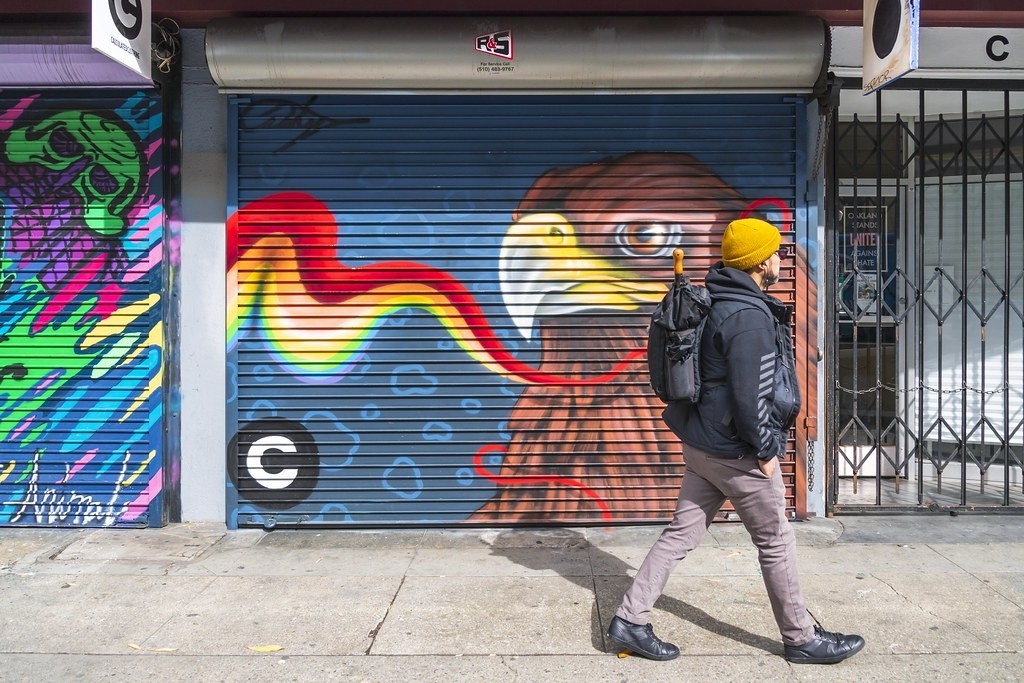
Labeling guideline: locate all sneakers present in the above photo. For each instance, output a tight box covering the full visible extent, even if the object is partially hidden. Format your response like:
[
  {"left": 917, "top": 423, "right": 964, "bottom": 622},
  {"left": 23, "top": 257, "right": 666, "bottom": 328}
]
[
  {"left": 607, "top": 616, "right": 680, "bottom": 662},
  {"left": 785, "top": 629, "right": 864, "bottom": 664}
]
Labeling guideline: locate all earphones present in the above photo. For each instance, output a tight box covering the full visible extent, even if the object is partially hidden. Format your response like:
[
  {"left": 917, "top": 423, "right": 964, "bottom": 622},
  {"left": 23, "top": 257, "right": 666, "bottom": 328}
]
[{"left": 761, "top": 261, "right": 766, "bottom": 267}]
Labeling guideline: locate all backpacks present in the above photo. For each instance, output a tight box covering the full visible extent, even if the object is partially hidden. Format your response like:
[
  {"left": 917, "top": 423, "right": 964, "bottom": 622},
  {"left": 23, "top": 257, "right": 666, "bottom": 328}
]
[{"left": 646, "top": 278, "right": 774, "bottom": 406}]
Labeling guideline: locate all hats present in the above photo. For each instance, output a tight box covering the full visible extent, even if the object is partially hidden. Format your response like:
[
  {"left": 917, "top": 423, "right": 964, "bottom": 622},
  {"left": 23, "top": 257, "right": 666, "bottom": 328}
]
[{"left": 721, "top": 219, "right": 781, "bottom": 271}]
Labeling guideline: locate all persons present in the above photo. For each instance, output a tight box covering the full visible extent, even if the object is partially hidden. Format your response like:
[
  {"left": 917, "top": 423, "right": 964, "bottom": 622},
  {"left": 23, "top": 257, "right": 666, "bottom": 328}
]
[{"left": 606, "top": 219, "right": 866, "bottom": 664}]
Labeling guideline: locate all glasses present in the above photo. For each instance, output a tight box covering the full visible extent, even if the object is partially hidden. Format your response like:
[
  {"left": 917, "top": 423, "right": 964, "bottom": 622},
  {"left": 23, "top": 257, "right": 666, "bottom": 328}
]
[{"left": 776, "top": 247, "right": 788, "bottom": 259}]
